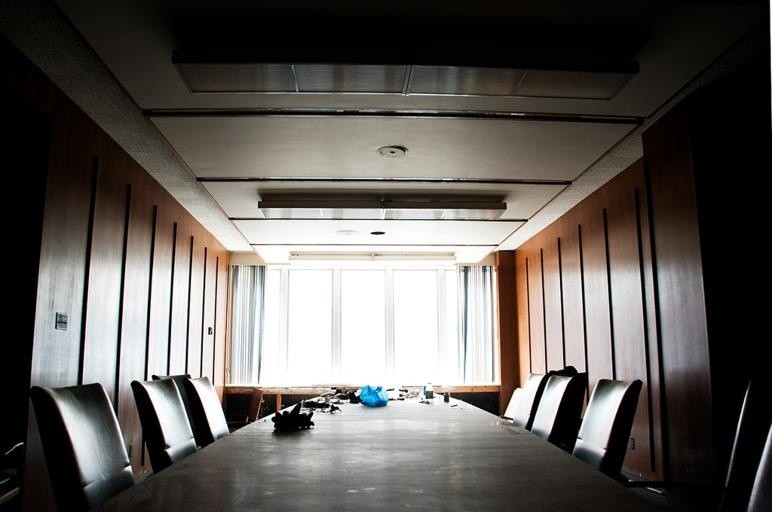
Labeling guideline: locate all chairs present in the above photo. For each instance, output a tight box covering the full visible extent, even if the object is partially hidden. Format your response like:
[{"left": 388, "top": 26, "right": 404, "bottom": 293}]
[
  {"left": 500, "top": 367, "right": 642, "bottom": 477},
  {"left": 30, "top": 373, "right": 282, "bottom": 511}
]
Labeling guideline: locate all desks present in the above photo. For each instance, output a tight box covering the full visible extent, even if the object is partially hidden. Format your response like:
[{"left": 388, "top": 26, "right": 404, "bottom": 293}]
[{"left": 99, "top": 388, "right": 661, "bottom": 512}]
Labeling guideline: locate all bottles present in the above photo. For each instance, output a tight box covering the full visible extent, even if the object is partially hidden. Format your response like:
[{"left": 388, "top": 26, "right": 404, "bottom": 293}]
[
  {"left": 425, "top": 382, "right": 434, "bottom": 402},
  {"left": 443, "top": 391, "right": 450, "bottom": 403}
]
